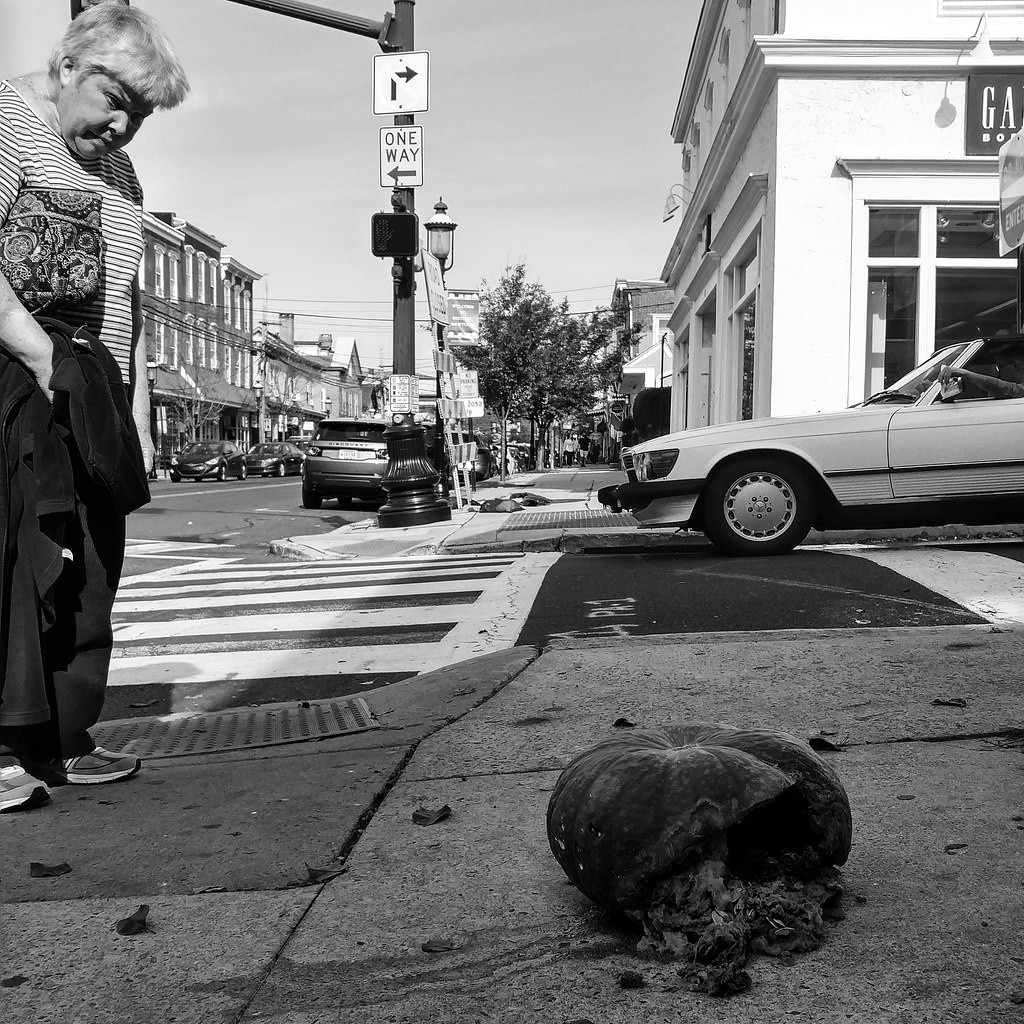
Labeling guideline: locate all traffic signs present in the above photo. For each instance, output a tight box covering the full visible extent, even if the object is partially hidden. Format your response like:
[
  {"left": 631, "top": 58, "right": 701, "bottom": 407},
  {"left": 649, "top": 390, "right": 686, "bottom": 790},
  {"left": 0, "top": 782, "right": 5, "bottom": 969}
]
[{"left": 380, "top": 124, "right": 423, "bottom": 188}]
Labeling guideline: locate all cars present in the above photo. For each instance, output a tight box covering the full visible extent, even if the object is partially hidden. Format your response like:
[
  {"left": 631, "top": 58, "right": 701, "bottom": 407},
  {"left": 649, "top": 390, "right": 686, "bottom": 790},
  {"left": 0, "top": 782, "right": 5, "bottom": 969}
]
[
  {"left": 300, "top": 417, "right": 389, "bottom": 508},
  {"left": 246, "top": 442, "right": 305, "bottom": 477},
  {"left": 595, "top": 333, "right": 1024, "bottom": 557},
  {"left": 169, "top": 440, "right": 248, "bottom": 482},
  {"left": 287, "top": 436, "right": 311, "bottom": 453},
  {"left": 447, "top": 430, "right": 496, "bottom": 484}
]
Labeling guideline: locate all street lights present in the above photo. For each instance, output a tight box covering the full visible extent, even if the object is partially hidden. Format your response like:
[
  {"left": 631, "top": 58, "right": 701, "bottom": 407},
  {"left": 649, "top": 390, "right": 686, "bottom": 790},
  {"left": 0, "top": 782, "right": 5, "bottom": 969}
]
[{"left": 423, "top": 193, "right": 458, "bottom": 500}]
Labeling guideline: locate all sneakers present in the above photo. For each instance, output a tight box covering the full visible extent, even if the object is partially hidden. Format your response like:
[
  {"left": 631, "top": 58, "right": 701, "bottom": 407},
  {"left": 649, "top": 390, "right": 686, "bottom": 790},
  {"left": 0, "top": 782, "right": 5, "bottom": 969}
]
[
  {"left": 21, "top": 747, "right": 141, "bottom": 784},
  {"left": 0, "top": 765, "right": 51, "bottom": 813}
]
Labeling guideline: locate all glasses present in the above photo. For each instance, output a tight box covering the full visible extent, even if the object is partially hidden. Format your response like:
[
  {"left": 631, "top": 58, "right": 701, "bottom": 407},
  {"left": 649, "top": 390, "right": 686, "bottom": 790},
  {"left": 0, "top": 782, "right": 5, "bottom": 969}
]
[{"left": 995, "top": 363, "right": 1012, "bottom": 372}]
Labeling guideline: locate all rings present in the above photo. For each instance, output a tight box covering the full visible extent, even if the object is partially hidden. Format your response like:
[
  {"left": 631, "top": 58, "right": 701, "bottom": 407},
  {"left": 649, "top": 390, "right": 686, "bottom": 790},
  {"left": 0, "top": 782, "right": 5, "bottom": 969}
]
[{"left": 944, "top": 373, "right": 947, "bottom": 375}]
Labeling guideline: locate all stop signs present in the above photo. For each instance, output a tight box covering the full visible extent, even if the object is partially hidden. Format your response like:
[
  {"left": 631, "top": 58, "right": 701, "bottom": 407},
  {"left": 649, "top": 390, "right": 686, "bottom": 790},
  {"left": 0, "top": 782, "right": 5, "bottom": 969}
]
[{"left": 998, "top": 128, "right": 1024, "bottom": 257}]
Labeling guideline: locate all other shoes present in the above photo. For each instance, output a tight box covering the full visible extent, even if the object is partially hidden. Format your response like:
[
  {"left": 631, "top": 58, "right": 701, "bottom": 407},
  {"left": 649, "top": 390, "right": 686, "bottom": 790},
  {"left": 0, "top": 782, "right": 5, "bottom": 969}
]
[
  {"left": 581, "top": 464, "right": 586, "bottom": 467},
  {"left": 567, "top": 466, "right": 572, "bottom": 467}
]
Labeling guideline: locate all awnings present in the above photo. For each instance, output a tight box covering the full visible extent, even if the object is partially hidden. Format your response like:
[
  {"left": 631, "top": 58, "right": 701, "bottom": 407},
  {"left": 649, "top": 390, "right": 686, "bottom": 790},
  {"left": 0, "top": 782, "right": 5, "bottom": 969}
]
[{"left": 147, "top": 363, "right": 272, "bottom": 415}]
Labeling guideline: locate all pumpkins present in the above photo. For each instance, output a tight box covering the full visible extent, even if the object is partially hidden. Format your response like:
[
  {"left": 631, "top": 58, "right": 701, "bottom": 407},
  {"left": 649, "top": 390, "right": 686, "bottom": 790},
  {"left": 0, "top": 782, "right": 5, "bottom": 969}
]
[{"left": 545, "top": 720, "right": 852, "bottom": 920}]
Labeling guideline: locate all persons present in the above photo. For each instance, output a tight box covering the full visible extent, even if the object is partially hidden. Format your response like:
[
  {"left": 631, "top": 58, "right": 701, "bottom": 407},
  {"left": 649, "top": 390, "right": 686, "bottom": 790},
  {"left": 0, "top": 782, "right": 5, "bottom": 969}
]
[
  {"left": 587, "top": 439, "right": 600, "bottom": 466},
  {"left": 915, "top": 343, "right": 1024, "bottom": 399},
  {"left": 489, "top": 446, "right": 563, "bottom": 478},
  {"left": 577, "top": 433, "right": 591, "bottom": 467},
  {"left": 0, "top": 0, "right": 190, "bottom": 813},
  {"left": 563, "top": 433, "right": 576, "bottom": 468}
]
